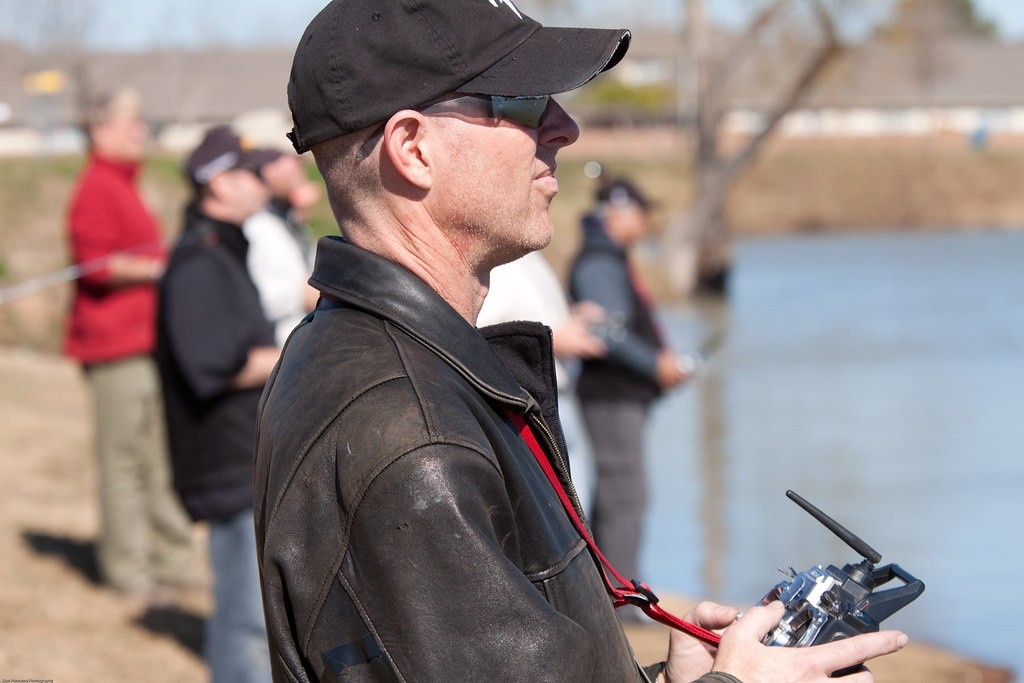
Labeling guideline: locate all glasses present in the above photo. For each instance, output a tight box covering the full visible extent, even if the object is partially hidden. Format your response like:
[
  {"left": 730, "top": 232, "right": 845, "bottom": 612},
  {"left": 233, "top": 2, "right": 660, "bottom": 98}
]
[{"left": 355, "top": 94, "right": 551, "bottom": 161}]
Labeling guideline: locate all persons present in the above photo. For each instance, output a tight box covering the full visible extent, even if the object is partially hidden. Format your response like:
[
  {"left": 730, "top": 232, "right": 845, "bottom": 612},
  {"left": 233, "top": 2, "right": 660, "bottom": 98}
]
[
  {"left": 559, "top": 179, "right": 688, "bottom": 617},
  {"left": 251, "top": 0, "right": 908, "bottom": 683},
  {"left": 475, "top": 246, "right": 610, "bottom": 549},
  {"left": 61, "top": 77, "right": 321, "bottom": 682}
]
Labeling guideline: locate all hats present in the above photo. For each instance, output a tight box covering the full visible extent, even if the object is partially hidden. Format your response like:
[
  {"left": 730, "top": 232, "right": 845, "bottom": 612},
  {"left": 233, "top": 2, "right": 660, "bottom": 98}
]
[
  {"left": 230, "top": 111, "right": 310, "bottom": 157},
  {"left": 286, "top": 0, "right": 632, "bottom": 155},
  {"left": 596, "top": 179, "right": 661, "bottom": 210},
  {"left": 184, "top": 127, "right": 285, "bottom": 184}
]
